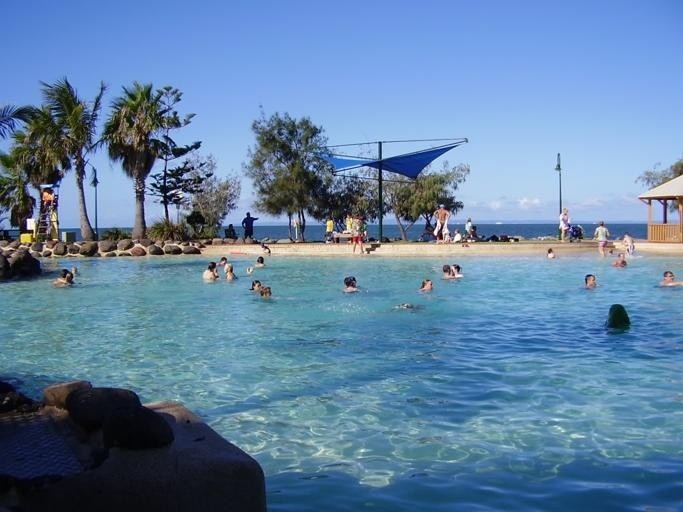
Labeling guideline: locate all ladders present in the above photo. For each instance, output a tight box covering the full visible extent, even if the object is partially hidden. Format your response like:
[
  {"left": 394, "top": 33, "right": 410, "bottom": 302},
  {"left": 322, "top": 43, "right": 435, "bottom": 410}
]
[{"left": 35, "top": 176, "right": 60, "bottom": 243}]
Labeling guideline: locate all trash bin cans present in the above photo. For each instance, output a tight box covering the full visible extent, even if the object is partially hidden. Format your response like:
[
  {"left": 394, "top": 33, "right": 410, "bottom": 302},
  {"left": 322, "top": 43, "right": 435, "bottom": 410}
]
[{"left": 62, "top": 231, "right": 76, "bottom": 242}]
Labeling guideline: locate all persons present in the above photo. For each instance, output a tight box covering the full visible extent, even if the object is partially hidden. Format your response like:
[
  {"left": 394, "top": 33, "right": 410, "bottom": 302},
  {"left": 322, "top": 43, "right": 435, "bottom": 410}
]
[
  {"left": 658, "top": 272, "right": 682, "bottom": 287},
  {"left": 344, "top": 276, "right": 357, "bottom": 290},
  {"left": 452, "top": 217, "right": 478, "bottom": 243},
  {"left": 240, "top": 212, "right": 259, "bottom": 239},
  {"left": 612, "top": 253, "right": 627, "bottom": 267},
  {"left": 593, "top": 221, "right": 610, "bottom": 257},
  {"left": 547, "top": 248, "right": 554, "bottom": 258},
  {"left": 443, "top": 264, "right": 463, "bottom": 277},
  {"left": 432, "top": 204, "right": 450, "bottom": 245},
  {"left": 226, "top": 224, "right": 238, "bottom": 239},
  {"left": 41, "top": 189, "right": 55, "bottom": 209},
  {"left": 619, "top": 234, "right": 637, "bottom": 256},
  {"left": 606, "top": 303, "right": 629, "bottom": 328},
  {"left": 54, "top": 269, "right": 74, "bottom": 287},
  {"left": 204, "top": 257, "right": 235, "bottom": 281},
  {"left": 421, "top": 279, "right": 432, "bottom": 289},
  {"left": 250, "top": 280, "right": 271, "bottom": 297},
  {"left": 558, "top": 208, "right": 570, "bottom": 243},
  {"left": 256, "top": 256, "right": 264, "bottom": 267},
  {"left": 325, "top": 215, "right": 366, "bottom": 253},
  {"left": 583, "top": 273, "right": 596, "bottom": 288}
]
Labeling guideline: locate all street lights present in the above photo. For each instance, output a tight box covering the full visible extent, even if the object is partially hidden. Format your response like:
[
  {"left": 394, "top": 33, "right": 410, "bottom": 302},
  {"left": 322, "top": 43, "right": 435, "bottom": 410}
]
[
  {"left": 174, "top": 190, "right": 182, "bottom": 224},
  {"left": 553, "top": 152, "right": 562, "bottom": 241},
  {"left": 89, "top": 166, "right": 99, "bottom": 240}
]
